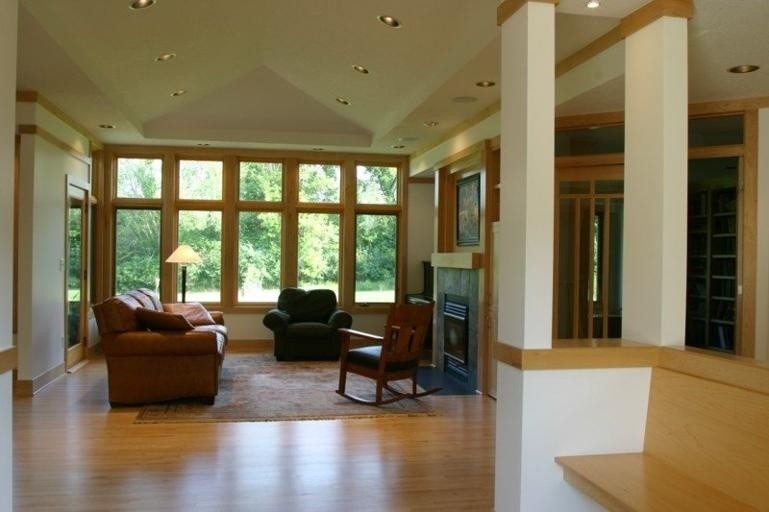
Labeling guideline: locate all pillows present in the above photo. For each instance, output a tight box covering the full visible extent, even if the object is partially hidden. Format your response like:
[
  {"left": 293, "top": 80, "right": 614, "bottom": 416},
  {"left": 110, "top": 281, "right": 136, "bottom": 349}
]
[
  {"left": 136, "top": 306, "right": 195, "bottom": 330},
  {"left": 162, "top": 301, "right": 215, "bottom": 325}
]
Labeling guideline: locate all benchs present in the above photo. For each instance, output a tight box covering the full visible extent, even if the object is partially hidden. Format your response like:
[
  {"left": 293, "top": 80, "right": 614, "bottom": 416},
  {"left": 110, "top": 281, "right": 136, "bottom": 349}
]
[{"left": 554, "top": 365, "right": 769, "bottom": 511}]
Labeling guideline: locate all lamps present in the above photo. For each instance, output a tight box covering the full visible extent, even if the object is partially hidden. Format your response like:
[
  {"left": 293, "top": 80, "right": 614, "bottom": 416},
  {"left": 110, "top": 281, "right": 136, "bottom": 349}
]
[{"left": 164, "top": 245, "right": 202, "bottom": 303}]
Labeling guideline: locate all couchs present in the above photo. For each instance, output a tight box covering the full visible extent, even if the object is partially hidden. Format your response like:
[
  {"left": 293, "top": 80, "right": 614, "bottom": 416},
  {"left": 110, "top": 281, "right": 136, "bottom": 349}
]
[{"left": 92, "top": 287, "right": 228, "bottom": 408}]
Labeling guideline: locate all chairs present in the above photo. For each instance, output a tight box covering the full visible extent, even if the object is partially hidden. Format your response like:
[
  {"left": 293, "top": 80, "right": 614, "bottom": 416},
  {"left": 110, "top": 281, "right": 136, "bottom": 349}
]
[
  {"left": 263, "top": 288, "right": 352, "bottom": 362},
  {"left": 335, "top": 300, "right": 443, "bottom": 406}
]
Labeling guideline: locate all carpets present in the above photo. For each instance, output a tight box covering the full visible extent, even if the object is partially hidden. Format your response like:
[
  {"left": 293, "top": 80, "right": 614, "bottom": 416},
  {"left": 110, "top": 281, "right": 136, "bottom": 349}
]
[
  {"left": 406, "top": 363, "right": 481, "bottom": 396},
  {"left": 132, "top": 356, "right": 437, "bottom": 425}
]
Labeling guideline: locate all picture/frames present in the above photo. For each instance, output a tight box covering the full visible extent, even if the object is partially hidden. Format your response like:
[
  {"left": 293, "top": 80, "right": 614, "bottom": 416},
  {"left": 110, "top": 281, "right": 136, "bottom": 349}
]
[{"left": 455, "top": 173, "right": 480, "bottom": 245}]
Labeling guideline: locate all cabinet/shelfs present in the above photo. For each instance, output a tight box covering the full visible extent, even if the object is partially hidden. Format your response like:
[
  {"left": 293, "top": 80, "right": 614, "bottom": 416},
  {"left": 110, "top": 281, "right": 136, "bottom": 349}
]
[{"left": 687, "top": 174, "right": 737, "bottom": 354}]
